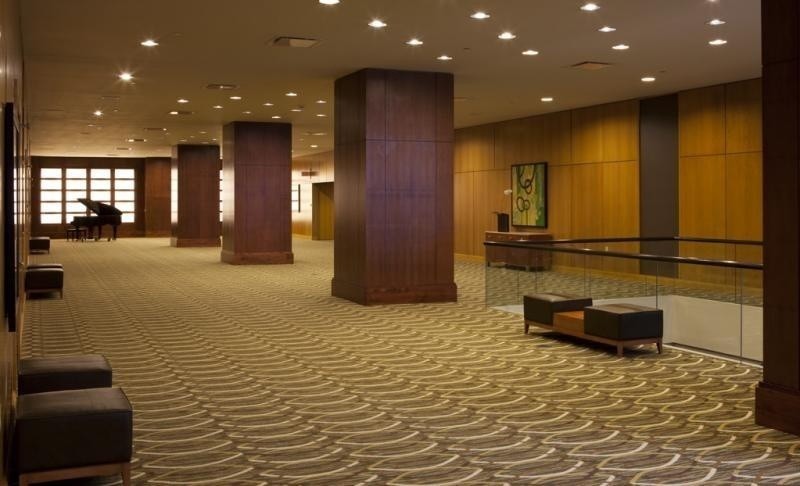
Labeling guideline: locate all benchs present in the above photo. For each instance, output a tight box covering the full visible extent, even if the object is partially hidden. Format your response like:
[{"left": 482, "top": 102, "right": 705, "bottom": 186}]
[
  {"left": 67, "top": 229, "right": 87, "bottom": 243},
  {"left": 519, "top": 290, "right": 665, "bottom": 356},
  {"left": 11, "top": 350, "right": 132, "bottom": 486},
  {"left": 27, "top": 262, "right": 65, "bottom": 299}
]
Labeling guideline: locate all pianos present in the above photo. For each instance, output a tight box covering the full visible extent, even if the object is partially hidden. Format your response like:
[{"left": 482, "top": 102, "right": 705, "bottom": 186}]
[{"left": 71, "top": 198, "right": 123, "bottom": 240}]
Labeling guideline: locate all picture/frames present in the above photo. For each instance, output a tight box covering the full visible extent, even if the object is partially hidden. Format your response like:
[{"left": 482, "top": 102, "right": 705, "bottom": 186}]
[
  {"left": 6, "top": 98, "right": 22, "bottom": 331},
  {"left": 510, "top": 162, "right": 550, "bottom": 229}
]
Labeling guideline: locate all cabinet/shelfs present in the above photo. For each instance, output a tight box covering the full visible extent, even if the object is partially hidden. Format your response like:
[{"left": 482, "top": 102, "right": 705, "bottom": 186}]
[{"left": 485, "top": 231, "right": 552, "bottom": 272}]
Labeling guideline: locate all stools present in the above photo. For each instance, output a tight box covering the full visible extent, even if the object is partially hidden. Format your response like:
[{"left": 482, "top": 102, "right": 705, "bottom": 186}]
[{"left": 29, "top": 236, "right": 50, "bottom": 253}]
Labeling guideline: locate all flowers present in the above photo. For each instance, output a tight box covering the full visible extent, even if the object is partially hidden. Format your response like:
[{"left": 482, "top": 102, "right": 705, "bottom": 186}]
[{"left": 492, "top": 188, "right": 512, "bottom": 214}]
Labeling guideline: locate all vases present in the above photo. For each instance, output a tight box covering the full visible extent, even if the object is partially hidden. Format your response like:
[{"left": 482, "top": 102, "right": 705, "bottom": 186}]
[{"left": 498, "top": 212, "right": 509, "bottom": 232}]
